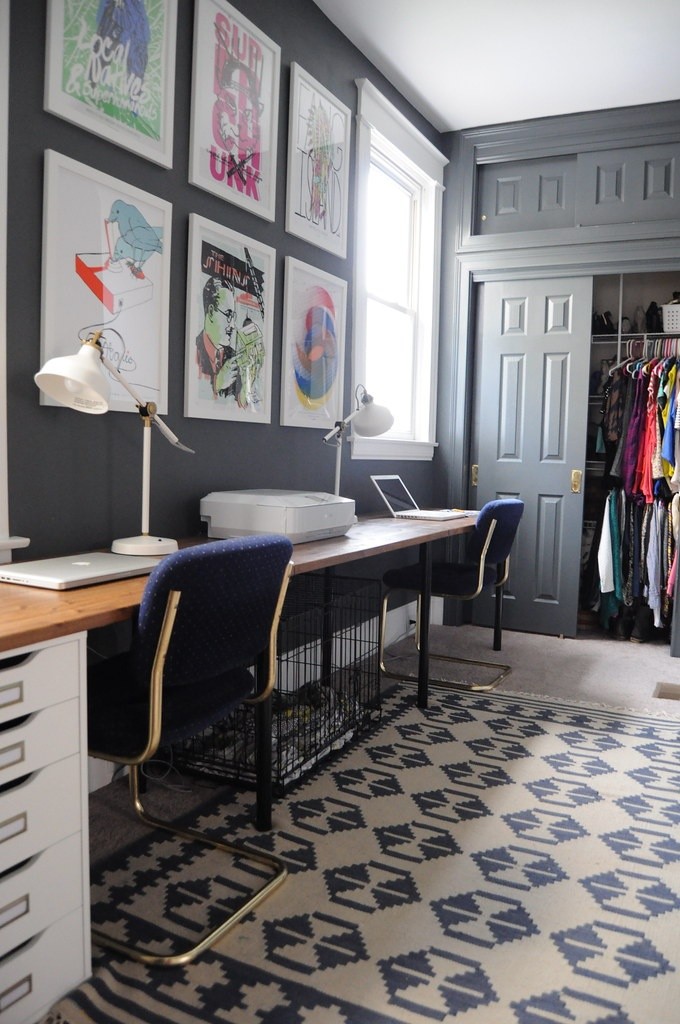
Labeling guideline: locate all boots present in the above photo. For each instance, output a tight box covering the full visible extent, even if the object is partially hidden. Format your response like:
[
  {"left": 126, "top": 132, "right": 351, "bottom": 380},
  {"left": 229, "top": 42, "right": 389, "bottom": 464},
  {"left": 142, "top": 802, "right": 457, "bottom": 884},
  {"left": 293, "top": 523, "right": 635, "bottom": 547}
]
[
  {"left": 613, "top": 604, "right": 633, "bottom": 640},
  {"left": 631, "top": 606, "right": 652, "bottom": 644}
]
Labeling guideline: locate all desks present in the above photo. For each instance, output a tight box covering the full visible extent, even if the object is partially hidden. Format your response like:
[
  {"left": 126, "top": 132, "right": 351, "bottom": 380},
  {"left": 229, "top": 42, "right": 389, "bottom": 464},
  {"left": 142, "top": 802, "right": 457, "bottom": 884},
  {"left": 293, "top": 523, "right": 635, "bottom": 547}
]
[{"left": 0, "top": 507, "right": 504, "bottom": 831}]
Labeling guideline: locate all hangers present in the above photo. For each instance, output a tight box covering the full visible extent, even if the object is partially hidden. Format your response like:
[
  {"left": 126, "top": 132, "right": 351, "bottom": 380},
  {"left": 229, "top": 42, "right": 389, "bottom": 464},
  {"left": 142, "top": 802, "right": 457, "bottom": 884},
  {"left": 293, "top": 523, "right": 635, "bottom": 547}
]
[{"left": 608, "top": 339, "right": 680, "bottom": 379}]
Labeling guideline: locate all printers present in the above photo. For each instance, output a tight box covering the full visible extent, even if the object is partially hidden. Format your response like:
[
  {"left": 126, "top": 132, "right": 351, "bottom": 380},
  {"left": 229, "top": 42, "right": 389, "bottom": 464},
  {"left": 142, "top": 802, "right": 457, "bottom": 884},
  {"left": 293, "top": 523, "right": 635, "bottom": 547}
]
[{"left": 200, "top": 489, "right": 355, "bottom": 545}]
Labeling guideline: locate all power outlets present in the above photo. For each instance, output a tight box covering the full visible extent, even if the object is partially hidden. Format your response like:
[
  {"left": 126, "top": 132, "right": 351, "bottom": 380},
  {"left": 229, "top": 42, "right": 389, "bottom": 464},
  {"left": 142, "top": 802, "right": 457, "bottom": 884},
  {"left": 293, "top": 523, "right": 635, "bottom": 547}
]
[{"left": 406, "top": 615, "right": 416, "bottom": 633}]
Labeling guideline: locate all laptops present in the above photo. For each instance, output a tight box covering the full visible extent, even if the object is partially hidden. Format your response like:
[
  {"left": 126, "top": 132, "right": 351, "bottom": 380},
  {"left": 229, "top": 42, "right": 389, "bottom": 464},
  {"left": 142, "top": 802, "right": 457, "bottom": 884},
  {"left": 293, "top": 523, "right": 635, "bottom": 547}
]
[
  {"left": 0, "top": 552, "right": 162, "bottom": 590},
  {"left": 370, "top": 475, "right": 466, "bottom": 521}
]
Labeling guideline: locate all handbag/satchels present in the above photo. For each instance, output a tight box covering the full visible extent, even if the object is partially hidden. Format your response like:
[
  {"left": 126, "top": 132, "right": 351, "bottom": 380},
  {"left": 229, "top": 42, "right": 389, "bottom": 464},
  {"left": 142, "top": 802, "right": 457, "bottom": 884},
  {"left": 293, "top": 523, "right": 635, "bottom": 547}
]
[
  {"left": 633, "top": 305, "right": 647, "bottom": 334},
  {"left": 659, "top": 299, "right": 680, "bottom": 334},
  {"left": 620, "top": 316, "right": 632, "bottom": 334},
  {"left": 592, "top": 310, "right": 618, "bottom": 335},
  {"left": 646, "top": 301, "right": 664, "bottom": 333}
]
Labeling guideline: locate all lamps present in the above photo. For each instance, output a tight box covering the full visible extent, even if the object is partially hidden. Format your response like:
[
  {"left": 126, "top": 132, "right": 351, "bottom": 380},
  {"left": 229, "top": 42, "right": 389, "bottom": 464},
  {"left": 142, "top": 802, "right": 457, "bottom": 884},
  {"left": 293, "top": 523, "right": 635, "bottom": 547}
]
[
  {"left": 322, "top": 384, "right": 395, "bottom": 524},
  {"left": 33, "top": 326, "right": 196, "bottom": 556}
]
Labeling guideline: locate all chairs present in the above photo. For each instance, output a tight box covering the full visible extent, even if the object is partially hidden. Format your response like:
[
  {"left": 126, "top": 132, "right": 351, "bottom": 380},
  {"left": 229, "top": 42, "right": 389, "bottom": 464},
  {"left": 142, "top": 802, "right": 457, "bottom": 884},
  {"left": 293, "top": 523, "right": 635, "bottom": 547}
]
[
  {"left": 377, "top": 496, "right": 524, "bottom": 692},
  {"left": 86, "top": 533, "right": 288, "bottom": 969}
]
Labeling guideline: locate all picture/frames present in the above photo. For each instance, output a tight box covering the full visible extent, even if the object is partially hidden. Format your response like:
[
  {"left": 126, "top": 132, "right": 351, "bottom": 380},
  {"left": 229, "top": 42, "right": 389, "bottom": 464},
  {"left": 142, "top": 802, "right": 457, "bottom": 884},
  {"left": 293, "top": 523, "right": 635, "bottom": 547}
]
[
  {"left": 43, "top": 0, "right": 178, "bottom": 171},
  {"left": 40, "top": 149, "right": 173, "bottom": 416},
  {"left": 183, "top": 212, "right": 276, "bottom": 424},
  {"left": 279, "top": 256, "right": 348, "bottom": 430},
  {"left": 284, "top": 61, "right": 351, "bottom": 259},
  {"left": 187, "top": 0, "right": 281, "bottom": 223}
]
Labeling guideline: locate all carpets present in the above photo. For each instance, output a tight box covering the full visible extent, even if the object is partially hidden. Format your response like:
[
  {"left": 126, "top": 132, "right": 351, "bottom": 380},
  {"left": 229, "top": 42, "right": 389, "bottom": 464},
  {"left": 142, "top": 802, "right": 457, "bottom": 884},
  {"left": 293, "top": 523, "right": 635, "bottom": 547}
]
[{"left": 36, "top": 672, "right": 680, "bottom": 1024}]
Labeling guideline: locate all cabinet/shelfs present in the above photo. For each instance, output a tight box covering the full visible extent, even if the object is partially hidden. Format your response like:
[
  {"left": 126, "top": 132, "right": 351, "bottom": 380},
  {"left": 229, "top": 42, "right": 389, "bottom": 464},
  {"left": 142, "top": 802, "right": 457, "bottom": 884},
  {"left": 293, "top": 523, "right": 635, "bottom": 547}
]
[
  {"left": 442, "top": 237, "right": 680, "bottom": 656},
  {"left": 0, "top": 630, "right": 93, "bottom": 1024},
  {"left": 454, "top": 102, "right": 680, "bottom": 253}
]
